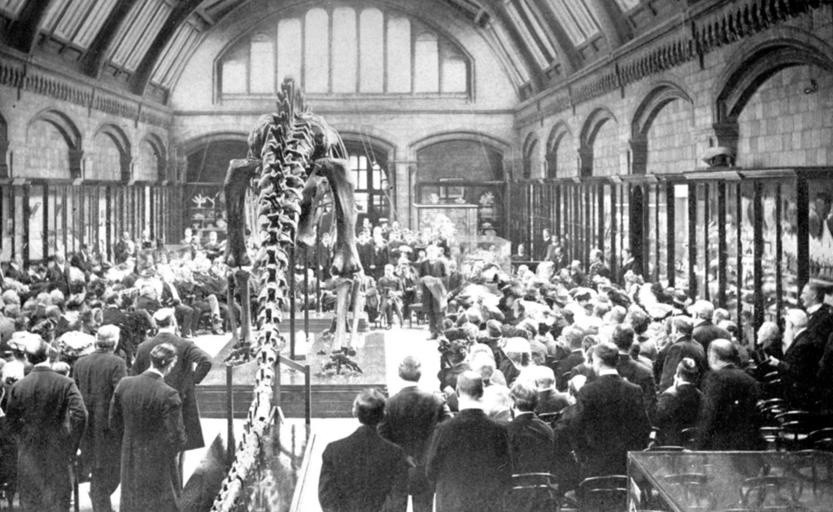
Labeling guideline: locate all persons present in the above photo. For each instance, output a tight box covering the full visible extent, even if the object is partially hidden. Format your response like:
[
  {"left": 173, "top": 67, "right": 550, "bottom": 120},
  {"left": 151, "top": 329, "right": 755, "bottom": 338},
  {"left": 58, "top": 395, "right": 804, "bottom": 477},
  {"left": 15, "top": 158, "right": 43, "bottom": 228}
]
[
  {"left": 2, "top": 333, "right": 88, "bottom": 511},
  {"left": 72, "top": 323, "right": 127, "bottom": 512},
  {"left": 305, "top": 212, "right": 833, "bottom": 457},
  {"left": 107, "top": 343, "right": 187, "bottom": 512},
  {"left": 0, "top": 214, "right": 243, "bottom": 413},
  {"left": 424, "top": 372, "right": 513, "bottom": 511},
  {"left": 318, "top": 388, "right": 408, "bottom": 511}
]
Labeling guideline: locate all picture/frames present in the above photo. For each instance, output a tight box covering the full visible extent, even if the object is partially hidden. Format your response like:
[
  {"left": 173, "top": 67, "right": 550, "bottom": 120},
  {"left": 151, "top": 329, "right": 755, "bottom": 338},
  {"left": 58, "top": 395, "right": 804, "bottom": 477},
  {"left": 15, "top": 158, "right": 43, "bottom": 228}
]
[{"left": 436, "top": 175, "right": 466, "bottom": 201}]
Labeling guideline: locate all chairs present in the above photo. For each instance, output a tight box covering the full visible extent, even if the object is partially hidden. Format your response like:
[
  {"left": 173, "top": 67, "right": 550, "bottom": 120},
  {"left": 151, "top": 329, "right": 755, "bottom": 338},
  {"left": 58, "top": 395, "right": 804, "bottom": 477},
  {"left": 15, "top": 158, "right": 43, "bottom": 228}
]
[
  {"left": 496, "top": 351, "right": 831, "bottom": 512},
  {"left": 183, "top": 272, "right": 446, "bottom": 333}
]
